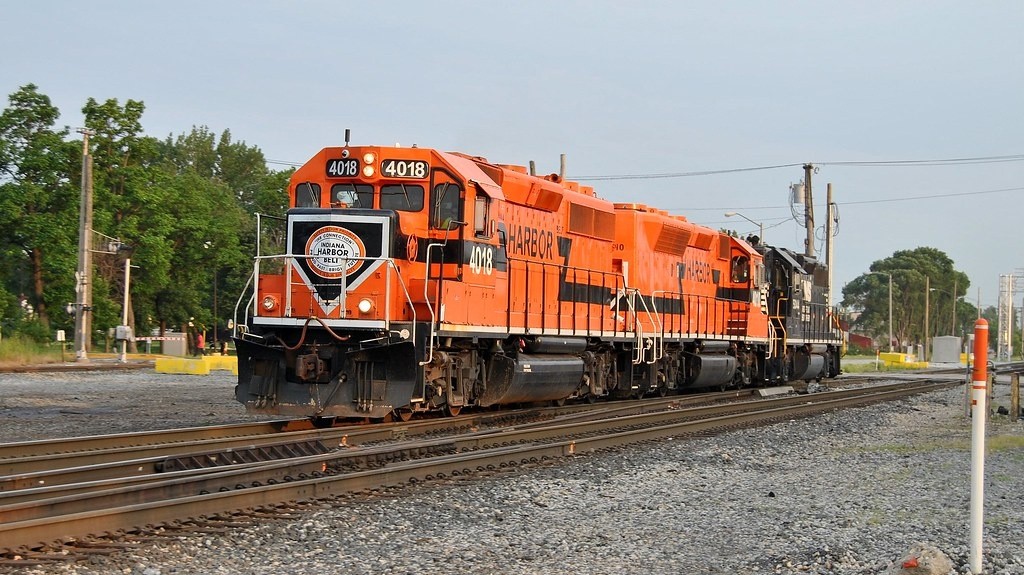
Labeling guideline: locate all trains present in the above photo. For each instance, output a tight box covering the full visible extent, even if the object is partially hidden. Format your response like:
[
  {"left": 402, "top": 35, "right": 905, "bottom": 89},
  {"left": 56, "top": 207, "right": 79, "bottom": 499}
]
[{"left": 230, "top": 128, "right": 846, "bottom": 427}]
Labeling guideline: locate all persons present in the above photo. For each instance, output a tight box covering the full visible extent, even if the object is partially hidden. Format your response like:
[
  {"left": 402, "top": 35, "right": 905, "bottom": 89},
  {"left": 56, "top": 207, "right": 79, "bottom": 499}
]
[
  {"left": 441, "top": 206, "right": 458, "bottom": 229},
  {"left": 193, "top": 327, "right": 230, "bottom": 357}
]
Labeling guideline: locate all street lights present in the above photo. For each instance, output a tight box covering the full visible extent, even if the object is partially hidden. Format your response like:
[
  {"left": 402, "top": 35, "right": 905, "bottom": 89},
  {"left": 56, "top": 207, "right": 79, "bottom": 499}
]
[
  {"left": 929, "top": 280, "right": 958, "bottom": 337},
  {"left": 725, "top": 212, "right": 764, "bottom": 246},
  {"left": 70, "top": 126, "right": 96, "bottom": 361},
  {"left": 862, "top": 270, "right": 893, "bottom": 352}
]
